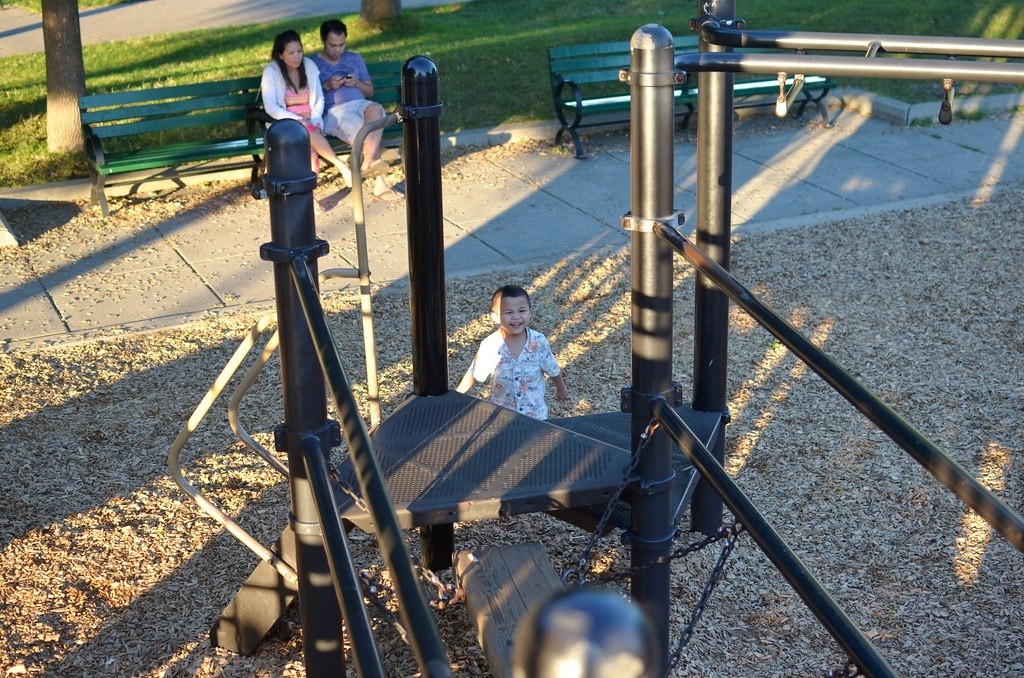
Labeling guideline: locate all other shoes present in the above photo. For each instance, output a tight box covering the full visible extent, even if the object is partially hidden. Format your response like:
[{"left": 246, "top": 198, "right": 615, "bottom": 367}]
[{"left": 341, "top": 162, "right": 353, "bottom": 187}]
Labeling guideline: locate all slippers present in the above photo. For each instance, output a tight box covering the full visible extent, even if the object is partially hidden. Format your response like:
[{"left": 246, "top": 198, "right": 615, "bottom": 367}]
[
  {"left": 369, "top": 189, "right": 405, "bottom": 204},
  {"left": 360, "top": 161, "right": 390, "bottom": 178}
]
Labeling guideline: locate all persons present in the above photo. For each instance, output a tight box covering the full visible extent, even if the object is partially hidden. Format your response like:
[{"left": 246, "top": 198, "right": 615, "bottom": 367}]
[
  {"left": 310, "top": 19, "right": 404, "bottom": 201},
  {"left": 456, "top": 286, "right": 568, "bottom": 421},
  {"left": 261, "top": 30, "right": 352, "bottom": 188}
]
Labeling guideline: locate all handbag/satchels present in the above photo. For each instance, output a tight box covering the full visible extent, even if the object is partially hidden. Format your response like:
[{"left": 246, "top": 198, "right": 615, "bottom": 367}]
[{"left": 245, "top": 64, "right": 277, "bottom": 123}]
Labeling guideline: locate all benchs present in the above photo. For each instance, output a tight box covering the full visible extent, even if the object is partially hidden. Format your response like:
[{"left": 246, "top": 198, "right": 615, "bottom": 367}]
[
  {"left": 548, "top": 33, "right": 848, "bottom": 158},
  {"left": 80, "top": 69, "right": 401, "bottom": 220}
]
